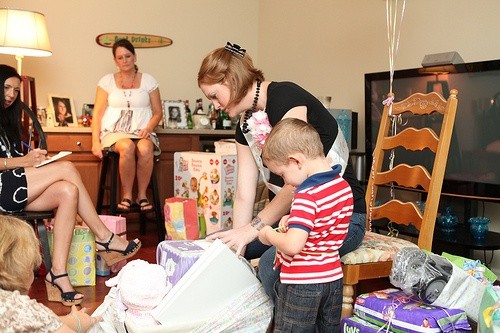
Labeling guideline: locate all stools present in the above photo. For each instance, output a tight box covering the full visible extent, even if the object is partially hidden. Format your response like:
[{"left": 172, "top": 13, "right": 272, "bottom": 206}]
[
  {"left": 157, "top": 240, "right": 204, "bottom": 294},
  {"left": 96, "top": 151, "right": 161, "bottom": 235}
]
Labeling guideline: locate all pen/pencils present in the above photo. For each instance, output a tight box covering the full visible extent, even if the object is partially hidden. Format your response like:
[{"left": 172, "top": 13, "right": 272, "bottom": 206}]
[{"left": 21, "top": 141, "right": 34, "bottom": 150}]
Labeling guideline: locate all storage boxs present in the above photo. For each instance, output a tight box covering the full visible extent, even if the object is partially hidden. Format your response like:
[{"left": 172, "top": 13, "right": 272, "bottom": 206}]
[
  {"left": 82, "top": 214, "right": 127, "bottom": 235},
  {"left": 174, "top": 139, "right": 261, "bottom": 236}
]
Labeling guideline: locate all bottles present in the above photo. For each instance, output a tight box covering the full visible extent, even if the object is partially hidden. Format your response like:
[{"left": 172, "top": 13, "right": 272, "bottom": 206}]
[
  {"left": 468, "top": 217, "right": 490, "bottom": 238},
  {"left": 437, "top": 205, "right": 458, "bottom": 232},
  {"left": 186, "top": 98, "right": 230, "bottom": 129}
]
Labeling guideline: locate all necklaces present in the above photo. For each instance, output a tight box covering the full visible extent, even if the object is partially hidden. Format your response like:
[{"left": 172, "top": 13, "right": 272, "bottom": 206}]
[
  {"left": 242, "top": 77, "right": 261, "bottom": 134},
  {"left": 120, "top": 70, "right": 137, "bottom": 108}
]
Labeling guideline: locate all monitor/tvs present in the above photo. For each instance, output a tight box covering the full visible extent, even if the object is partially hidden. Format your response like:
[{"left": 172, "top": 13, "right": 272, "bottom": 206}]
[{"left": 364, "top": 59, "right": 500, "bottom": 204}]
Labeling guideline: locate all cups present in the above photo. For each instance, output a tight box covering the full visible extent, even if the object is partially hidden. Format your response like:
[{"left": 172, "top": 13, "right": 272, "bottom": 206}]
[{"left": 317, "top": 96, "right": 331, "bottom": 109}]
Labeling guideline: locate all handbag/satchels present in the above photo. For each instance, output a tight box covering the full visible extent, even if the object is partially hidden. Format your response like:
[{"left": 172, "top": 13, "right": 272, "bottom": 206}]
[
  {"left": 47, "top": 227, "right": 96, "bottom": 286},
  {"left": 81, "top": 215, "right": 127, "bottom": 276},
  {"left": 413, "top": 249, "right": 487, "bottom": 322},
  {"left": 164, "top": 197, "right": 199, "bottom": 241}
]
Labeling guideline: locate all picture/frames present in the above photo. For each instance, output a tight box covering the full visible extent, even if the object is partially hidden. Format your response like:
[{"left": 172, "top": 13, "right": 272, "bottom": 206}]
[
  {"left": 49, "top": 93, "right": 77, "bottom": 128},
  {"left": 161, "top": 100, "right": 188, "bottom": 129}
]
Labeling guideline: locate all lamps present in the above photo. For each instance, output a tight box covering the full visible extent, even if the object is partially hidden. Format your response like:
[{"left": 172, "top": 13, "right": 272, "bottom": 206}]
[{"left": 0, "top": 6, "right": 52, "bottom": 122}]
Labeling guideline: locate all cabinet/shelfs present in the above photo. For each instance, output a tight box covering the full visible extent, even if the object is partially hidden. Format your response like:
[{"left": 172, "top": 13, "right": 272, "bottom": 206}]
[
  {"left": 346, "top": 175, "right": 500, "bottom": 260},
  {"left": 34, "top": 129, "right": 200, "bottom": 225}
]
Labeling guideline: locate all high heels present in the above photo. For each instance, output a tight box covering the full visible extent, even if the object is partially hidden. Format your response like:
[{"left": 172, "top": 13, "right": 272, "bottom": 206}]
[
  {"left": 94, "top": 232, "right": 142, "bottom": 267},
  {"left": 44, "top": 268, "right": 83, "bottom": 306}
]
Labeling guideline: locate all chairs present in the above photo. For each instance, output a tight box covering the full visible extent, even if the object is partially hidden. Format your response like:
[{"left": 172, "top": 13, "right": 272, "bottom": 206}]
[
  {"left": 334, "top": 88, "right": 459, "bottom": 321},
  {"left": 10, "top": 101, "right": 54, "bottom": 276}
]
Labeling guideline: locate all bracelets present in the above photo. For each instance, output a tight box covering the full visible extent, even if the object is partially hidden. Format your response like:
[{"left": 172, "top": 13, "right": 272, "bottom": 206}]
[
  {"left": 251, "top": 216, "right": 265, "bottom": 231},
  {"left": 5, "top": 158, "right": 7, "bottom": 169},
  {"left": 69, "top": 314, "right": 81, "bottom": 332}
]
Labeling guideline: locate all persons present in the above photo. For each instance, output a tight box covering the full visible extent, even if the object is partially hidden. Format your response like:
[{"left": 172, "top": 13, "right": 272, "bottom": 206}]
[
  {"left": 212, "top": 169, "right": 219, "bottom": 178},
  {"left": 197, "top": 41, "right": 366, "bottom": 301},
  {"left": 92, "top": 38, "right": 163, "bottom": 212},
  {"left": 0, "top": 64, "right": 141, "bottom": 306},
  {"left": 185, "top": 177, "right": 201, "bottom": 211},
  {"left": 54, "top": 99, "right": 72, "bottom": 123},
  {"left": 0, "top": 215, "right": 104, "bottom": 333},
  {"left": 257, "top": 118, "right": 354, "bottom": 333},
  {"left": 210, "top": 212, "right": 218, "bottom": 224},
  {"left": 169, "top": 107, "right": 181, "bottom": 122},
  {"left": 213, "top": 189, "right": 219, "bottom": 200}
]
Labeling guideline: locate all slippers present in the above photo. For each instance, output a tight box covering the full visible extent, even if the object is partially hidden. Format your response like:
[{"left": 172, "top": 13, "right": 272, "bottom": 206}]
[
  {"left": 118, "top": 198, "right": 132, "bottom": 213},
  {"left": 136, "top": 198, "right": 153, "bottom": 212}
]
